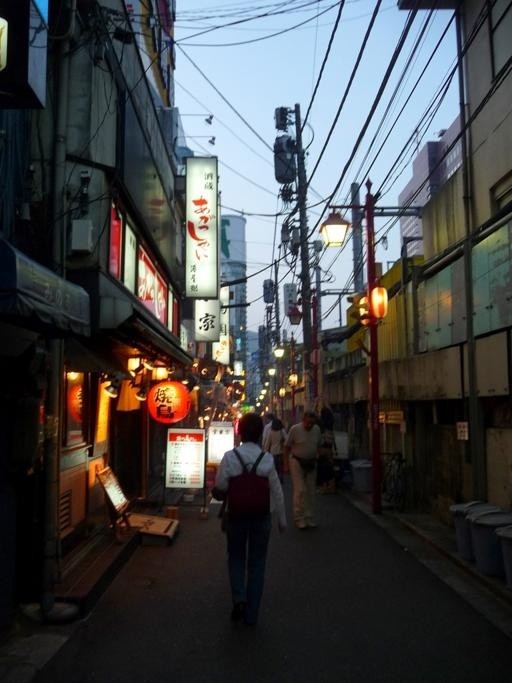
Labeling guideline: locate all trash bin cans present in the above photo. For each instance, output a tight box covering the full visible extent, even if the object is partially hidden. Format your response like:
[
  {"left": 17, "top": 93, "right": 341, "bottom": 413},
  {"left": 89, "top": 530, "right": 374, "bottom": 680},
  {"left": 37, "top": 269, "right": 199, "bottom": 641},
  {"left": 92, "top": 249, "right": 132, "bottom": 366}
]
[
  {"left": 449, "top": 503, "right": 511, "bottom": 580},
  {"left": 351, "top": 460, "right": 374, "bottom": 503}
]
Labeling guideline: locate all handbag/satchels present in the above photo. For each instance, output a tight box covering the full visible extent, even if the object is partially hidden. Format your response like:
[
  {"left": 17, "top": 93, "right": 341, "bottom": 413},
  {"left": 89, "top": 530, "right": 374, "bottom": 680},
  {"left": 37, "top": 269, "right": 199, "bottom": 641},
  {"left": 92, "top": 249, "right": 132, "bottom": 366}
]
[{"left": 292, "top": 453, "right": 317, "bottom": 472}]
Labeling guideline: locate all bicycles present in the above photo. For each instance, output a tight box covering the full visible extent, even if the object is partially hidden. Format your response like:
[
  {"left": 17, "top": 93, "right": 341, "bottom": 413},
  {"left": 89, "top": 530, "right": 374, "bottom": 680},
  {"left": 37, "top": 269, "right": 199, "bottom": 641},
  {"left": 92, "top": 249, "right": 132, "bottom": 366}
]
[{"left": 377, "top": 450, "right": 410, "bottom": 512}]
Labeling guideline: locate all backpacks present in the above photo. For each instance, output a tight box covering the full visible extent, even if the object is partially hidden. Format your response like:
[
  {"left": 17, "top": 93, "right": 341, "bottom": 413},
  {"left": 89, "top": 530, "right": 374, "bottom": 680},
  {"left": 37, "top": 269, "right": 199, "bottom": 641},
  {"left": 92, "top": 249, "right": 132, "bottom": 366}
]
[{"left": 221, "top": 449, "right": 272, "bottom": 531}]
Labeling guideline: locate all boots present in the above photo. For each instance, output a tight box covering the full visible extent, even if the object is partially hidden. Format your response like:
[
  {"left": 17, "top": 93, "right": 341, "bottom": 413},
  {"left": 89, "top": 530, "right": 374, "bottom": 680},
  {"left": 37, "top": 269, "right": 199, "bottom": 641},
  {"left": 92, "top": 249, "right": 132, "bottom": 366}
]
[
  {"left": 230, "top": 602, "right": 245, "bottom": 626},
  {"left": 240, "top": 605, "right": 255, "bottom": 633}
]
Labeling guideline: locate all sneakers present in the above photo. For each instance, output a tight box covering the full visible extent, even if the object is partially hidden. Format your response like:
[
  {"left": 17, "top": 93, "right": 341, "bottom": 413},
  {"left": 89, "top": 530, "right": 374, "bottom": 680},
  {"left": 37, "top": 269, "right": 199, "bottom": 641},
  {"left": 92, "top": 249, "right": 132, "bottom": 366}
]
[
  {"left": 306, "top": 518, "right": 320, "bottom": 528},
  {"left": 295, "top": 519, "right": 308, "bottom": 529}
]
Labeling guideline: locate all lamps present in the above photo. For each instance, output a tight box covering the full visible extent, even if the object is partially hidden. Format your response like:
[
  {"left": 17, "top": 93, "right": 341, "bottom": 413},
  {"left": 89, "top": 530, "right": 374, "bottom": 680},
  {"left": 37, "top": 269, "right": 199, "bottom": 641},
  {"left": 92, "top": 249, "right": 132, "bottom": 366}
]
[
  {"left": 127, "top": 357, "right": 169, "bottom": 381},
  {"left": 68, "top": 385, "right": 85, "bottom": 425}
]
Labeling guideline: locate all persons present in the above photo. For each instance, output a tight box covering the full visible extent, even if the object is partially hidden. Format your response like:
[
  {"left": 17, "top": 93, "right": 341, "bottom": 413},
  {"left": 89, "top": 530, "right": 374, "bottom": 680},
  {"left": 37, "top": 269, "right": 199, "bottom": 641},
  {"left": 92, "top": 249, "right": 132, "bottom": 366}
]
[
  {"left": 282, "top": 409, "right": 325, "bottom": 530},
  {"left": 214, "top": 410, "right": 287, "bottom": 626},
  {"left": 258, "top": 409, "right": 333, "bottom": 498}
]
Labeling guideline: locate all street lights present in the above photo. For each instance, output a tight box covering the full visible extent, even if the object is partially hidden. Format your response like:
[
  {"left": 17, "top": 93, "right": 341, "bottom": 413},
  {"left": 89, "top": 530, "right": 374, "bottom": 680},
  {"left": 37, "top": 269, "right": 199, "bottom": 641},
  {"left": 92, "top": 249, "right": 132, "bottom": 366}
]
[
  {"left": 254, "top": 287, "right": 322, "bottom": 473},
  {"left": 319, "top": 176, "right": 386, "bottom": 515}
]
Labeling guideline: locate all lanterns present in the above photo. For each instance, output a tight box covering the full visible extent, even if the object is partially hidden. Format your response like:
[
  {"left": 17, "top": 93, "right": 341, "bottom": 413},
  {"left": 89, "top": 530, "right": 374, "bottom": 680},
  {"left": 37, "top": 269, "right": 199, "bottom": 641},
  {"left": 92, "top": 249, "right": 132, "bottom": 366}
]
[
  {"left": 370, "top": 284, "right": 389, "bottom": 320},
  {"left": 279, "top": 387, "right": 286, "bottom": 397},
  {"left": 291, "top": 373, "right": 297, "bottom": 384},
  {"left": 288, "top": 375, "right": 294, "bottom": 386},
  {"left": 358, "top": 296, "right": 370, "bottom": 325}
]
